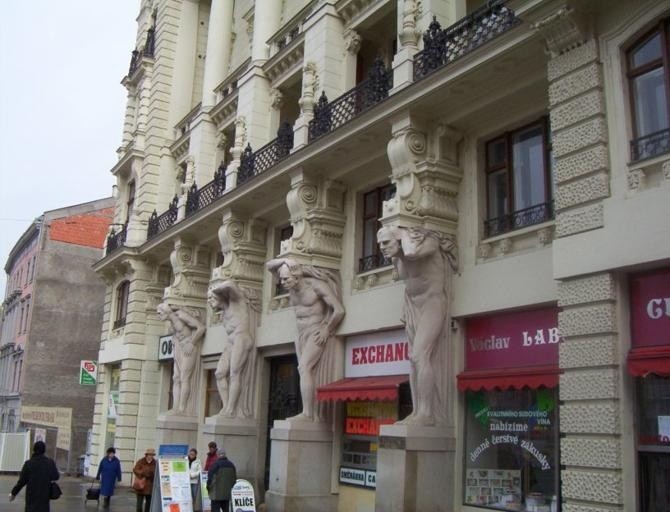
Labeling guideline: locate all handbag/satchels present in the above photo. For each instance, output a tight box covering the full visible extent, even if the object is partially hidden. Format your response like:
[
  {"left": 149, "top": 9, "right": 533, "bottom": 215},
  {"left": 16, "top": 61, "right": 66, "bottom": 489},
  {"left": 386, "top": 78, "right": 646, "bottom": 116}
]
[
  {"left": 50, "top": 482, "right": 61, "bottom": 500},
  {"left": 133, "top": 475, "right": 146, "bottom": 491}
]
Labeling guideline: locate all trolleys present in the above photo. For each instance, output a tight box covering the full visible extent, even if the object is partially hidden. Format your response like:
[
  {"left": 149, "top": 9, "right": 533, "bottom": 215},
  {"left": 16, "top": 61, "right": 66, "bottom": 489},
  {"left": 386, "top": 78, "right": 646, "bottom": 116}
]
[{"left": 82, "top": 478, "right": 102, "bottom": 505}]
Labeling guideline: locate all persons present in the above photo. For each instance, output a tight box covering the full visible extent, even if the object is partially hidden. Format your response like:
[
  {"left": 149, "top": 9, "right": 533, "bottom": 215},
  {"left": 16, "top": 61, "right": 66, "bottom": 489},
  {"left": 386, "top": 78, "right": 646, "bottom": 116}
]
[
  {"left": 95, "top": 446, "right": 124, "bottom": 512},
  {"left": 206, "top": 447, "right": 238, "bottom": 512},
  {"left": 185, "top": 447, "right": 203, "bottom": 502},
  {"left": 156, "top": 301, "right": 207, "bottom": 420},
  {"left": 7, "top": 440, "right": 61, "bottom": 512},
  {"left": 202, "top": 440, "right": 221, "bottom": 472},
  {"left": 132, "top": 447, "right": 157, "bottom": 512},
  {"left": 259, "top": 257, "right": 347, "bottom": 426},
  {"left": 205, "top": 278, "right": 255, "bottom": 421},
  {"left": 375, "top": 226, "right": 447, "bottom": 431}
]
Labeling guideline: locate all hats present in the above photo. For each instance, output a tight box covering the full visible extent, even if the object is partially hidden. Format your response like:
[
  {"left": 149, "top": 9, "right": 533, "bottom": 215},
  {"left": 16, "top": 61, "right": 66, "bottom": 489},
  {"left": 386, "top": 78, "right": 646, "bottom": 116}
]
[{"left": 145, "top": 448, "right": 156, "bottom": 456}]
